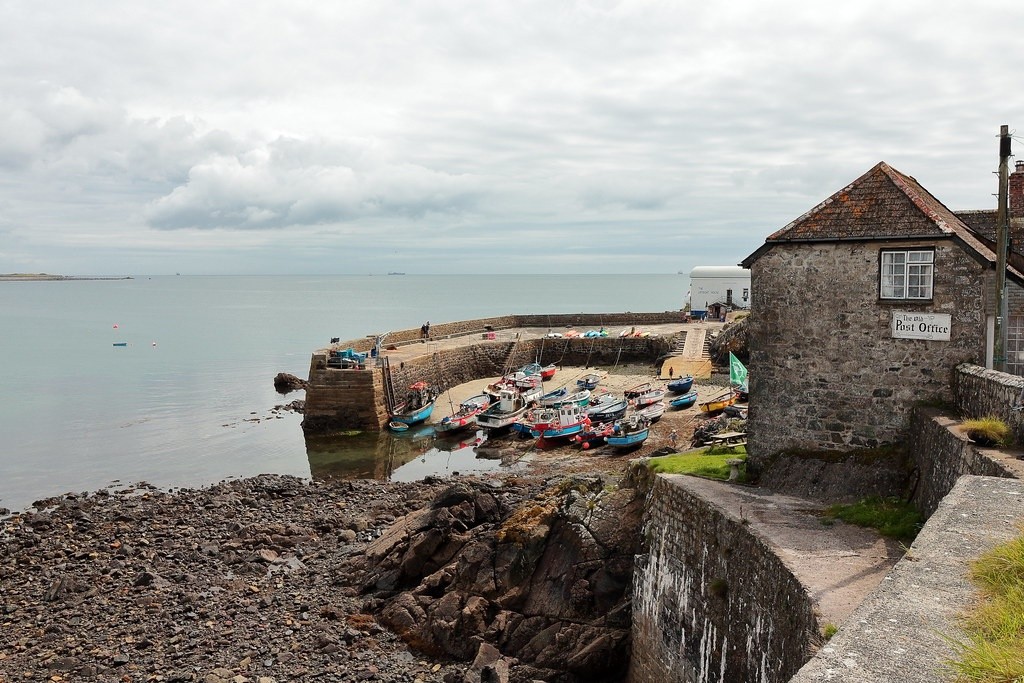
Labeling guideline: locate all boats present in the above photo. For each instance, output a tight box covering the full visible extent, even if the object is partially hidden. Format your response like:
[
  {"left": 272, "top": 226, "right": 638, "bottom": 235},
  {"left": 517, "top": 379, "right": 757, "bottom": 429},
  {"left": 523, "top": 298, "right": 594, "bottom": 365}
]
[
  {"left": 669, "top": 391, "right": 699, "bottom": 409},
  {"left": 433, "top": 394, "right": 492, "bottom": 432},
  {"left": 723, "top": 405, "right": 748, "bottom": 417},
  {"left": 392, "top": 380, "right": 437, "bottom": 425},
  {"left": 633, "top": 403, "right": 667, "bottom": 422},
  {"left": 474, "top": 387, "right": 528, "bottom": 428},
  {"left": 632, "top": 389, "right": 665, "bottom": 407},
  {"left": 698, "top": 390, "right": 737, "bottom": 414},
  {"left": 734, "top": 383, "right": 749, "bottom": 400},
  {"left": 623, "top": 382, "right": 652, "bottom": 396},
  {"left": 482, "top": 356, "right": 651, "bottom": 453},
  {"left": 666, "top": 375, "right": 695, "bottom": 393},
  {"left": 389, "top": 421, "right": 410, "bottom": 431}
]
